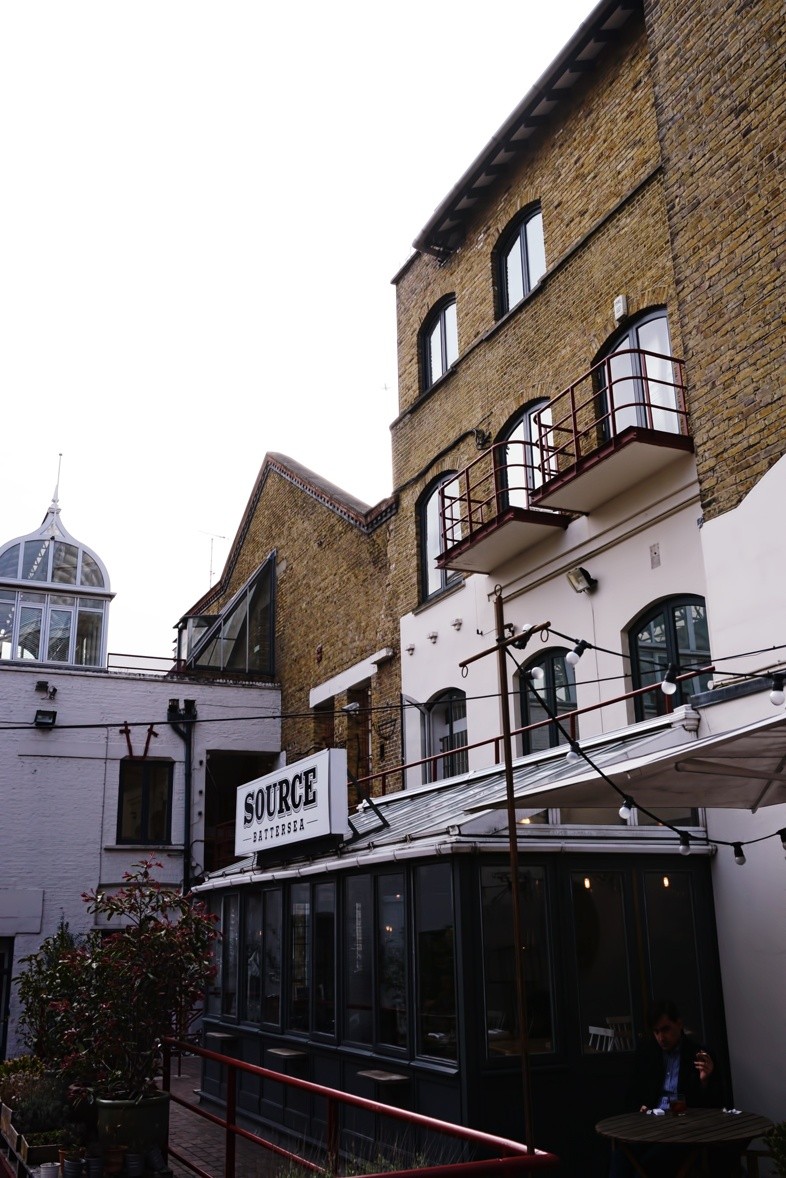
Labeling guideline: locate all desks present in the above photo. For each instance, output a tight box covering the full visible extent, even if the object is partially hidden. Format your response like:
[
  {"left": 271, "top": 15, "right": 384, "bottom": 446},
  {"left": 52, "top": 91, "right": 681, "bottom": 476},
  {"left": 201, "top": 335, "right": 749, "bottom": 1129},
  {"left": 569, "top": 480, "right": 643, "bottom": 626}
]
[
  {"left": 488, "top": 1038, "right": 596, "bottom": 1056},
  {"left": 595, "top": 1108, "right": 775, "bottom": 1178},
  {"left": 423, "top": 1030, "right": 457, "bottom": 1057}
]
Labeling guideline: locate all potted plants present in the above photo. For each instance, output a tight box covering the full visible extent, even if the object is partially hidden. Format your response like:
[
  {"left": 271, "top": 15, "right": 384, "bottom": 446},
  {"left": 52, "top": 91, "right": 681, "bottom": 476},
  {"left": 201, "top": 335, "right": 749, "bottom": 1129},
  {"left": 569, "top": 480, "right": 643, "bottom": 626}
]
[{"left": 0, "top": 851, "right": 231, "bottom": 1178}]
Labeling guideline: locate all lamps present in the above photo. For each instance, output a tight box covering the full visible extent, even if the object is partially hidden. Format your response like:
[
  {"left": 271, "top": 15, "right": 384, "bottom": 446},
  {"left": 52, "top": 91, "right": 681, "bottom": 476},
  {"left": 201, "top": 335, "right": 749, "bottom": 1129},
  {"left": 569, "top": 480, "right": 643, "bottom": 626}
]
[
  {"left": 566, "top": 567, "right": 598, "bottom": 595},
  {"left": 34, "top": 710, "right": 57, "bottom": 731},
  {"left": 48, "top": 685, "right": 57, "bottom": 699}
]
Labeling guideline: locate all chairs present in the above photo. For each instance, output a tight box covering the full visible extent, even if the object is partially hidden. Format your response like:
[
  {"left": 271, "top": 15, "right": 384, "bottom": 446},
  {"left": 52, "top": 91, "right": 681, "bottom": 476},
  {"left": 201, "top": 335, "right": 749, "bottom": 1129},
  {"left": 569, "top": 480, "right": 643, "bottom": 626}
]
[
  {"left": 588, "top": 1015, "right": 635, "bottom": 1052},
  {"left": 486, "top": 1010, "right": 536, "bottom": 1036}
]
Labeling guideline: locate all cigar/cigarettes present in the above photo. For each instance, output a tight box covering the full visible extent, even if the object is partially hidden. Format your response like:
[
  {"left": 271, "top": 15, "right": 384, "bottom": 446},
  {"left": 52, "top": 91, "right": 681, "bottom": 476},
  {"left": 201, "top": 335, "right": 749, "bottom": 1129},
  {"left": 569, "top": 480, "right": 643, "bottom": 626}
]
[{"left": 698, "top": 1052, "right": 708, "bottom": 1055}]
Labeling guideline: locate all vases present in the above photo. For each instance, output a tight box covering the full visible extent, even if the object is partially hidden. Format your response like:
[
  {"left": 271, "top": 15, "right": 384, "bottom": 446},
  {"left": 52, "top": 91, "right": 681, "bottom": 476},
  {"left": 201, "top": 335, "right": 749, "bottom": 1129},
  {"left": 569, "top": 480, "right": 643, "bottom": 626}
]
[
  {"left": 40, "top": 1162, "right": 61, "bottom": 1178},
  {"left": 146, "top": 1143, "right": 166, "bottom": 1171}
]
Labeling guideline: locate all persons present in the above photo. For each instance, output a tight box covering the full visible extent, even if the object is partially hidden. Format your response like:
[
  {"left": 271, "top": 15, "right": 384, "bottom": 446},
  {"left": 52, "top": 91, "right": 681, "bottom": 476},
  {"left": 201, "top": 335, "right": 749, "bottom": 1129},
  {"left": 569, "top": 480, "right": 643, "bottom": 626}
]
[{"left": 627, "top": 1004, "right": 720, "bottom": 1112}]
